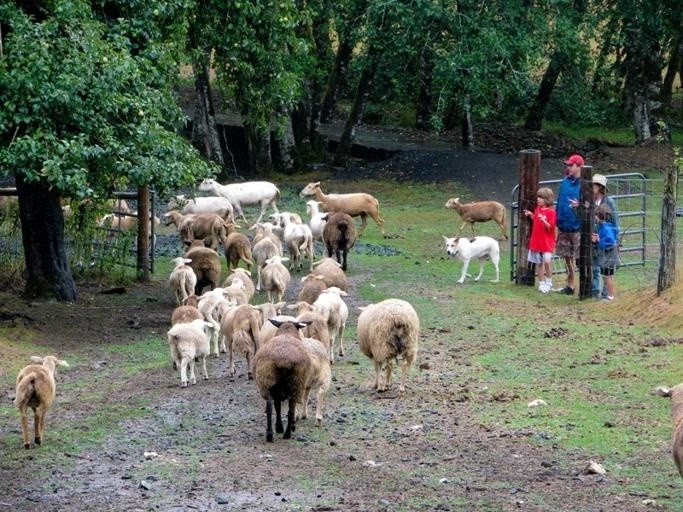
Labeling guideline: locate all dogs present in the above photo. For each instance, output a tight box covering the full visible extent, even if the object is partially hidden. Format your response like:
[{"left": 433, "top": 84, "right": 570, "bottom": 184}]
[{"left": 441, "top": 233, "right": 502, "bottom": 284}]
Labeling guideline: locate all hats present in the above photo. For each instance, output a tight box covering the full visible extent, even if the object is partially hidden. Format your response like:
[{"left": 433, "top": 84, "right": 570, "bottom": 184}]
[
  {"left": 591, "top": 173, "right": 609, "bottom": 192},
  {"left": 564, "top": 155, "right": 584, "bottom": 166}
]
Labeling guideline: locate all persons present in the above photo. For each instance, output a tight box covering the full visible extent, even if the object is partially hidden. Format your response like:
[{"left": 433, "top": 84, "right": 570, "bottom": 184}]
[
  {"left": 569, "top": 173, "right": 619, "bottom": 300},
  {"left": 555, "top": 155, "right": 586, "bottom": 294},
  {"left": 592, "top": 204, "right": 620, "bottom": 299},
  {"left": 522, "top": 188, "right": 557, "bottom": 294}
]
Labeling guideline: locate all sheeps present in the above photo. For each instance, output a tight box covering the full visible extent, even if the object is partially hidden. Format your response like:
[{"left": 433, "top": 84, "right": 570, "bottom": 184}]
[
  {"left": 0, "top": 196, "right": 21, "bottom": 226},
  {"left": 252, "top": 318, "right": 312, "bottom": 441},
  {"left": 162, "top": 175, "right": 356, "bottom": 389},
  {"left": 11, "top": 354, "right": 71, "bottom": 449},
  {"left": 445, "top": 198, "right": 510, "bottom": 241},
  {"left": 300, "top": 181, "right": 384, "bottom": 234},
  {"left": 355, "top": 299, "right": 419, "bottom": 397},
  {"left": 285, "top": 320, "right": 331, "bottom": 426},
  {"left": 60, "top": 196, "right": 160, "bottom": 257}
]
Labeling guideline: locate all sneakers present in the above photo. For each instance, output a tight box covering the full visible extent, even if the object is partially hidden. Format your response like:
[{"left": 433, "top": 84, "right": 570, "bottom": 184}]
[
  {"left": 592, "top": 296, "right": 614, "bottom": 301},
  {"left": 538, "top": 278, "right": 575, "bottom": 295}
]
